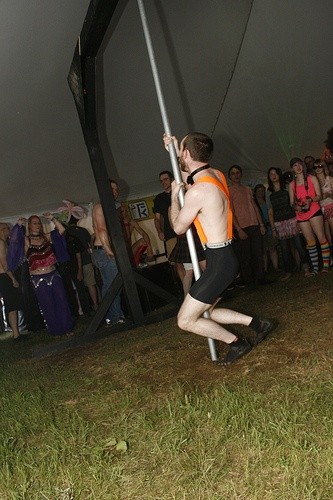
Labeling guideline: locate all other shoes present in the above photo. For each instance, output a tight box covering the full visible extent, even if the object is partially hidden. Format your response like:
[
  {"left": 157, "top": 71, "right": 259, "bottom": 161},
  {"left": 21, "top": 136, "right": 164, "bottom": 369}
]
[
  {"left": 12, "top": 333, "right": 32, "bottom": 342},
  {"left": 226, "top": 277, "right": 249, "bottom": 291},
  {"left": 105, "top": 316, "right": 127, "bottom": 328}
]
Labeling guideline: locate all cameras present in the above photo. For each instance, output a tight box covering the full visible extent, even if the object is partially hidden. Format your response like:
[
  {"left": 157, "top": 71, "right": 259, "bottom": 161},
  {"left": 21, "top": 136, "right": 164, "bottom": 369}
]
[{"left": 299, "top": 204, "right": 309, "bottom": 212}]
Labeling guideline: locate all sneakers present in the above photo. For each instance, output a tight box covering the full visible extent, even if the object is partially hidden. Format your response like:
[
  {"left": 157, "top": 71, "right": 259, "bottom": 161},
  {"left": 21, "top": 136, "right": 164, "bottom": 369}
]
[
  {"left": 223, "top": 336, "right": 252, "bottom": 366},
  {"left": 248, "top": 316, "right": 280, "bottom": 346}
]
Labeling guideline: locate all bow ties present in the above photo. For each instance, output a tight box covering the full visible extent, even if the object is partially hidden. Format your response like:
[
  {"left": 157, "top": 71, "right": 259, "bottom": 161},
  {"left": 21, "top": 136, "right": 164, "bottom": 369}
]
[{"left": 185, "top": 164, "right": 211, "bottom": 186}]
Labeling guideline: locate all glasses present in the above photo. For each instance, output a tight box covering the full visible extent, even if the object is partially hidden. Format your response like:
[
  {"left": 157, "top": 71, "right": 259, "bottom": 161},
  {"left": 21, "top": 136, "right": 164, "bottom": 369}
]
[{"left": 312, "top": 164, "right": 324, "bottom": 170}]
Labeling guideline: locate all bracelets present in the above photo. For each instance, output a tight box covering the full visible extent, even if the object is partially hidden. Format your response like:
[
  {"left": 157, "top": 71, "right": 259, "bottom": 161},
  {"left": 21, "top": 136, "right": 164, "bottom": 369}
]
[{"left": 235, "top": 224, "right": 242, "bottom": 232}]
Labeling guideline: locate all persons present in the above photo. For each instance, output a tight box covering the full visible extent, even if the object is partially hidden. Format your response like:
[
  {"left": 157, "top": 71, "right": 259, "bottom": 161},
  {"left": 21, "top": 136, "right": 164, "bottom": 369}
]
[
  {"left": 250, "top": 144, "right": 333, "bottom": 279},
  {"left": 153, "top": 171, "right": 185, "bottom": 283},
  {"left": 0, "top": 176, "right": 153, "bottom": 343},
  {"left": 160, "top": 124, "right": 279, "bottom": 365},
  {"left": 227, "top": 163, "right": 273, "bottom": 287},
  {"left": 169, "top": 223, "right": 209, "bottom": 299}
]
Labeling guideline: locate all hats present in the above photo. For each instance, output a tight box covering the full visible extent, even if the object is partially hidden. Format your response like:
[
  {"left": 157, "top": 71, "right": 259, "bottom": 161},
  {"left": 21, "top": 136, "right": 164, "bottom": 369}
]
[{"left": 289, "top": 157, "right": 303, "bottom": 167}]
